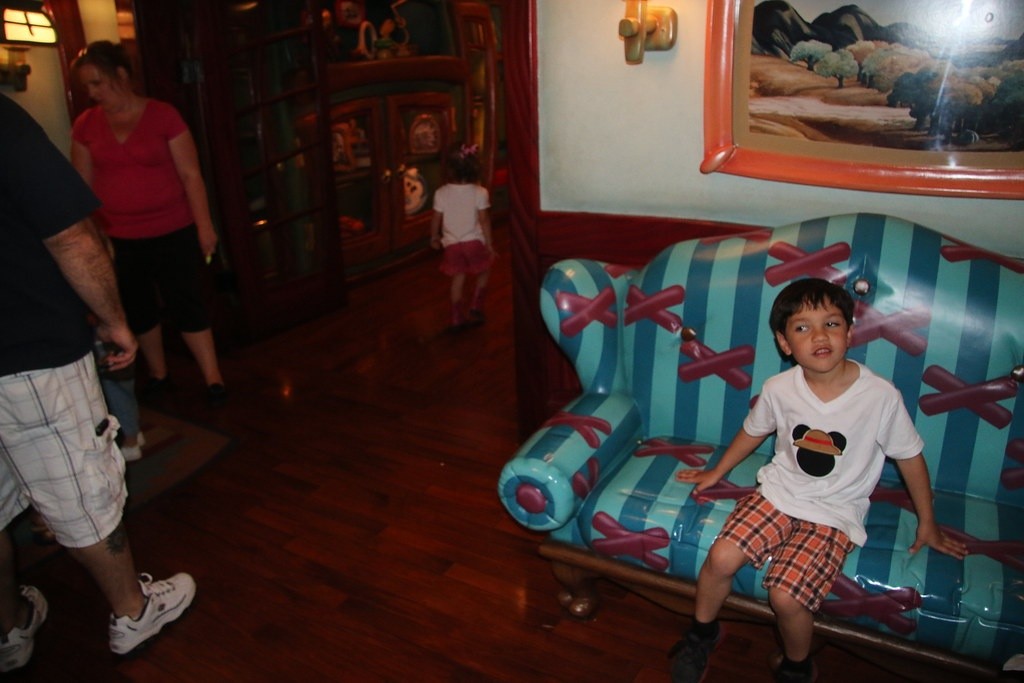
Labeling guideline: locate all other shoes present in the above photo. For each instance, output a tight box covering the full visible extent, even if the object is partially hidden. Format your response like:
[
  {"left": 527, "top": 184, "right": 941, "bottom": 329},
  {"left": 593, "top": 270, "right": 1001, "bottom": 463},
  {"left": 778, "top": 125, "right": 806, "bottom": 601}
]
[
  {"left": 205, "top": 382, "right": 229, "bottom": 406},
  {"left": 142, "top": 376, "right": 171, "bottom": 400}
]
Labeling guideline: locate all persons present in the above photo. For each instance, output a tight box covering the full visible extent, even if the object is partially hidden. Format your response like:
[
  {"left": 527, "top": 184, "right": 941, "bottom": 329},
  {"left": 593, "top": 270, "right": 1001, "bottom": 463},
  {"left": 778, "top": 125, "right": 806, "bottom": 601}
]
[
  {"left": 665, "top": 278, "right": 972, "bottom": 683},
  {"left": 65, "top": 38, "right": 225, "bottom": 415},
  {"left": 428, "top": 151, "right": 497, "bottom": 329},
  {"left": 0, "top": 92, "right": 197, "bottom": 671}
]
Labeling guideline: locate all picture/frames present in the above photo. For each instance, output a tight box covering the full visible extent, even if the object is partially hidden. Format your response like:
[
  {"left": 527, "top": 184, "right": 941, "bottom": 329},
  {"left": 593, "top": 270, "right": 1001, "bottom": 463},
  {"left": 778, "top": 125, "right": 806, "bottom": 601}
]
[{"left": 699, "top": 1, "right": 1023, "bottom": 201}]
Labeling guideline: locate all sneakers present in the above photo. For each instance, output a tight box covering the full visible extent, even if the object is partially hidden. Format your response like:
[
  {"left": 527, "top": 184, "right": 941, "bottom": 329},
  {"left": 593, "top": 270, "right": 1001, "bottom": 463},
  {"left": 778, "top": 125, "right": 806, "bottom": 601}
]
[
  {"left": 668, "top": 622, "right": 724, "bottom": 682},
  {"left": 108, "top": 571, "right": 198, "bottom": 653},
  {"left": 0, "top": 586, "right": 49, "bottom": 670},
  {"left": 776, "top": 656, "right": 819, "bottom": 683}
]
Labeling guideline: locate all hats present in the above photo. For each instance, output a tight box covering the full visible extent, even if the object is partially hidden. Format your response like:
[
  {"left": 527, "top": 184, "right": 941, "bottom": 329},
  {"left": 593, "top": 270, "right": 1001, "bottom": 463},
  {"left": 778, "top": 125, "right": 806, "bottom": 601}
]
[{"left": 447, "top": 146, "right": 484, "bottom": 177}]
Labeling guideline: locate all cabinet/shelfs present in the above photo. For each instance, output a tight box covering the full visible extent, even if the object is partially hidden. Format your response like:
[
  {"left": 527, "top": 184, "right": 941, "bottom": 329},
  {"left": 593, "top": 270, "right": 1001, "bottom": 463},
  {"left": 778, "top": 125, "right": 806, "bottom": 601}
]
[{"left": 137, "top": 1, "right": 339, "bottom": 357}]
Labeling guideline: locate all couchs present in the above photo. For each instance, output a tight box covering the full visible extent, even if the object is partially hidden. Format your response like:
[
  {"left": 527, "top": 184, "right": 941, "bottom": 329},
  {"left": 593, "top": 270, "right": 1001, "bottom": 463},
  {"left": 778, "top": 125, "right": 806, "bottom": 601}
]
[{"left": 498, "top": 212, "right": 1023, "bottom": 683}]
